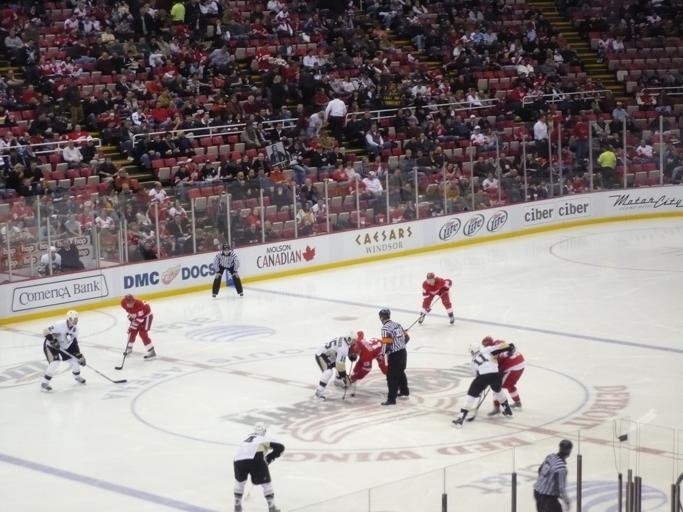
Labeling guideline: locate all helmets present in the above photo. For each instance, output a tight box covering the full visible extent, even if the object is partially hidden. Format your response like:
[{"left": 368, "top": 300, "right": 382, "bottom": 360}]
[
  {"left": 427, "top": 273, "right": 434, "bottom": 279},
  {"left": 379, "top": 309, "right": 390, "bottom": 318},
  {"left": 67, "top": 311, "right": 79, "bottom": 327},
  {"left": 559, "top": 440, "right": 572, "bottom": 448},
  {"left": 482, "top": 336, "right": 493, "bottom": 346},
  {"left": 351, "top": 344, "right": 361, "bottom": 353},
  {"left": 125, "top": 294, "right": 135, "bottom": 303},
  {"left": 254, "top": 422, "right": 265, "bottom": 433}
]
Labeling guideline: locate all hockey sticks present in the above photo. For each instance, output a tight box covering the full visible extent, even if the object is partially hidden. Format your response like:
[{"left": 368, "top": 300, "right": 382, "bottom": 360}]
[
  {"left": 467, "top": 386, "right": 491, "bottom": 421},
  {"left": 115, "top": 332, "right": 131, "bottom": 369},
  {"left": 403, "top": 293, "right": 443, "bottom": 332},
  {"left": 342, "top": 362, "right": 356, "bottom": 400},
  {"left": 46, "top": 342, "right": 126, "bottom": 383}
]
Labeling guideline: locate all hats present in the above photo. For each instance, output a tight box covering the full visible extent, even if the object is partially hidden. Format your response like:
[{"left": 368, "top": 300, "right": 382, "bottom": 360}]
[{"left": 223, "top": 244, "right": 231, "bottom": 251}]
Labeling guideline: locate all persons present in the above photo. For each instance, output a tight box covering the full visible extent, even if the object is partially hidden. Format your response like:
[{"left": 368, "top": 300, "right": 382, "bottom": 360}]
[
  {"left": 532, "top": 438, "right": 573, "bottom": 512},
  {"left": 482, "top": 335, "right": 526, "bottom": 415},
  {"left": 41, "top": 310, "right": 86, "bottom": 393},
  {"left": 211, "top": 243, "right": 243, "bottom": 297},
  {"left": 453, "top": 342, "right": 515, "bottom": 423},
  {"left": 0, "top": 0, "right": 682, "bottom": 278},
  {"left": 314, "top": 308, "right": 410, "bottom": 406},
  {"left": 417, "top": 273, "right": 454, "bottom": 324},
  {"left": 230, "top": 423, "right": 284, "bottom": 512},
  {"left": 121, "top": 295, "right": 156, "bottom": 360}
]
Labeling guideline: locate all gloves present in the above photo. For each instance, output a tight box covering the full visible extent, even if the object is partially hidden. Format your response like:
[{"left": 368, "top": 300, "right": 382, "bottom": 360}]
[
  {"left": 51, "top": 338, "right": 60, "bottom": 350},
  {"left": 77, "top": 354, "right": 85, "bottom": 366}
]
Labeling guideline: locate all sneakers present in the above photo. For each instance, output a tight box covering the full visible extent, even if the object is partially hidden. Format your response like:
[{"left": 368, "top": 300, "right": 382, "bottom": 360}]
[
  {"left": 510, "top": 403, "right": 521, "bottom": 407},
  {"left": 75, "top": 377, "right": 85, "bottom": 383},
  {"left": 144, "top": 347, "right": 155, "bottom": 358},
  {"left": 450, "top": 318, "right": 454, "bottom": 324},
  {"left": 41, "top": 383, "right": 52, "bottom": 390},
  {"left": 381, "top": 400, "right": 396, "bottom": 405},
  {"left": 489, "top": 407, "right": 500, "bottom": 415},
  {"left": 502, "top": 411, "right": 512, "bottom": 415},
  {"left": 453, "top": 419, "right": 463, "bottom": 425},
  {"left": 123, "top": 347, "right": 132, "bottom": 355}
]
